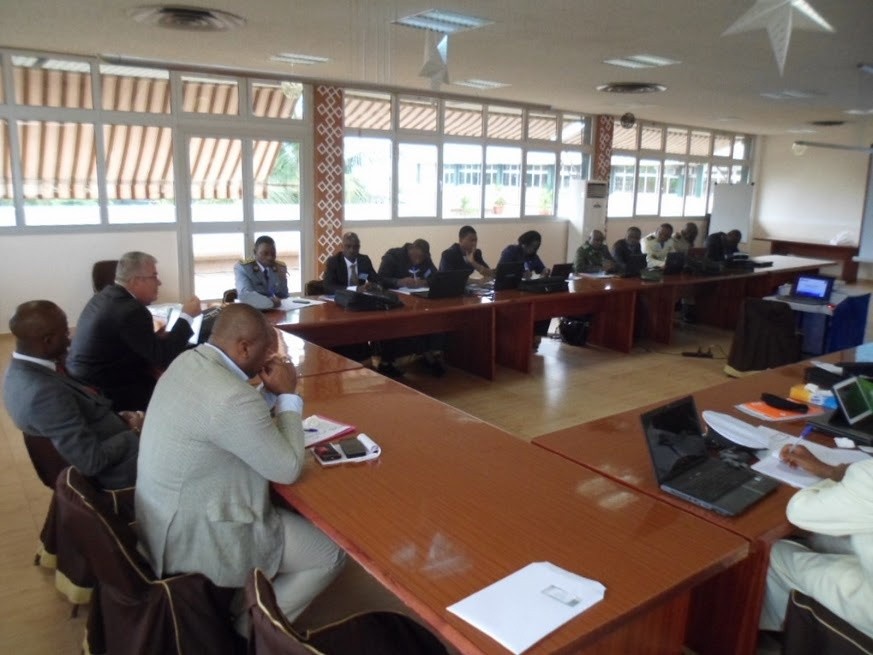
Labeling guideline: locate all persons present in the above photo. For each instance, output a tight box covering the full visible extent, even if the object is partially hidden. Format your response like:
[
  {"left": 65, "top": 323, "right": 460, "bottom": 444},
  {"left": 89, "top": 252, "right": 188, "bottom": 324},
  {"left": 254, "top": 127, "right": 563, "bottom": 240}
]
[
  {"left": 376, "top": 238, "right": 442, "bottom": 292},
  {"left": 574, "top": 229, "right": 617, "bottom": 272},
  {"left": 670, "top": 222, "right": 698, "bottom": 261},
  {"left": 703, "top": 228, "right": 743, "bottom": 261},
  {"left": 1, "top": 299, "right": 148, "bottom": 488},
  {"left": 64, "top": 248, "right": 203, "bottom": 424},
  {"left": 639, "top": 223, "right": 681, "bottom": 273},
  {"left": 755, "top": 442, "right": 873, "bottom": 654},
  {"left": 232, "top": 235, "right": 288, "bottom": 311},
  {"left": 610, "top": 227, "right": 648, "bottom": 272},
  {"left": 435, "top": 225, "right": 495, "bottom": 293},
  {"left": 134, "top": 302, "right": 344, "bottom": 643},
  {"left": 322, "top": 232, "right": 383, "bottom": 297},
  {"left": 492, "top": 229, "right": 551, "bottom": 288}
]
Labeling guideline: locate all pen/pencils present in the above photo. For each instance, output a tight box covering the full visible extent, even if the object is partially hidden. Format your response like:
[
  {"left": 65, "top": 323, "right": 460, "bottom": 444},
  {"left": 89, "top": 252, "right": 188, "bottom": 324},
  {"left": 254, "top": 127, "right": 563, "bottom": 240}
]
[
  {"left": 786, "top": 423, "right": 813, "bottom": 468},
  {"left": 303, "top": 429, "right": 317, "bottom": 433}
]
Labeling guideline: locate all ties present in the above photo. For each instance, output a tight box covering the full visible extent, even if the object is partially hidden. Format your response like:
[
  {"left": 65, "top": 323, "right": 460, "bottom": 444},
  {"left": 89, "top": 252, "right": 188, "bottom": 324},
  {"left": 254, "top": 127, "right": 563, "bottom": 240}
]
[
  {"left": 263, "top": 270, "right": 269, "bottom": 283},
  {"left": 349, "top": 265, "right": 358, "bottom": 285},
  {"left": 54, "top": 365, "right": 96, "bottom": 396}
]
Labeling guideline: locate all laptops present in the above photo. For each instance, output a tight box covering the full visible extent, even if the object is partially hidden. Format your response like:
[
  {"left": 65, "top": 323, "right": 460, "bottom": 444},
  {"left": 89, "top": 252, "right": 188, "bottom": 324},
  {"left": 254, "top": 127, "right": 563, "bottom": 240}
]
[
  {"left": 410, "top": 268, "right": 469, "bottom": 300},
  {"left": 682, "top": 247, "right": 708, "bottom": 268},
  {"left": 651, "top": 252, "right": 684, "bottom": 275},
  {"left": 162, "top": 307, "right": 204, "bottom": 349},
  {"left": 777, "top": 270, "right": 836, "bottom": 306},
  {"left": 604, "top": 254, "right": 646, "bottom": 278},
  {"left": 549, "top": 263, "right": 573, "bottom": 284},
  {"left": 638, "top": 395, "right": 780, "bottom": 520},
  {"left": 476, "top": 262, "right": 525, "bottom": 291}
]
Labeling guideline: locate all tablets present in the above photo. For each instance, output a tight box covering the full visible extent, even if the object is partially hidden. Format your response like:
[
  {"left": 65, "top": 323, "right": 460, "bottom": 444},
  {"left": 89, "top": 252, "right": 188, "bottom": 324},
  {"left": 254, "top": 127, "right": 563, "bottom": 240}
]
[{"left": 834, "top": 375, "right": 872, "bottom": 425}]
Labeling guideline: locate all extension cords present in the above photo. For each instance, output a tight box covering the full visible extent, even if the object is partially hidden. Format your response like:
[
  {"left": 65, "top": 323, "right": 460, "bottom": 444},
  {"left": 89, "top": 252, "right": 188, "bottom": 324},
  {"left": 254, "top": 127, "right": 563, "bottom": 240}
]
[{"left": 682, "top": 348, "right": 713, "bottom": 358}]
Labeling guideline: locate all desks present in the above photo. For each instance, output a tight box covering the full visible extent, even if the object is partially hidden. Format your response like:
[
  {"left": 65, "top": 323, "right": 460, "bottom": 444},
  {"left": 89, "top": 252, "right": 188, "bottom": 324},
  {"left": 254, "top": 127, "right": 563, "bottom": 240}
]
[
  {"left": 634, "top": 253, "right": 837, "bottom": 348},
  {"left": 137, "top": 313, "right": 365, "bottom": 386},
  {"left": 268, "top": 367, "right": 749, "bottom": 655},
  {"left": 772, "top": 239, "right": 859, "bottom": 282},
  {"left": 493, "top": 271, "right": 645, "bottom": 375},
  {"left": 255, "top": 280, "right": 511, "bottom": 385},
  {"left": 535, "top": 340, "right": 872, "bottom": 654}
]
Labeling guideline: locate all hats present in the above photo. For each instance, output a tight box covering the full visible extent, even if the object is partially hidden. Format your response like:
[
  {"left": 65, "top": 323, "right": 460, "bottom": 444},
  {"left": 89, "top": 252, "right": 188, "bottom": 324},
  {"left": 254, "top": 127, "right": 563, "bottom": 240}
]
[{"left": 702, "top": 410, "right": 770, "bottom": 454}]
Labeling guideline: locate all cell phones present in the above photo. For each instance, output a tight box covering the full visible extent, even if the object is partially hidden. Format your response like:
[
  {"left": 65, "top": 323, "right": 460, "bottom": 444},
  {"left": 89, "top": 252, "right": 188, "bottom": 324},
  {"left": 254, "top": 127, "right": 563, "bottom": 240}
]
[
  {"left": 339, "top": 437, "right": 365, "bottom": 458},
  {"left": 293, "top": 299, "right": 310, "bottom": 305},
  {"left": 315, "top": 444, "right": 342, "bottom": 462}
]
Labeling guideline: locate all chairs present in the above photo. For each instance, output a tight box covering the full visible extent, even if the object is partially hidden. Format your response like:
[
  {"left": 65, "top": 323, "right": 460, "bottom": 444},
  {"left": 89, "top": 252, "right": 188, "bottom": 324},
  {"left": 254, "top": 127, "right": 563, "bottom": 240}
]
[
  {"left": 246, "top": 568, "right": 448, "bottom": 655},
  {"left": 782, "top": 590, "right": 872, "bottom": 654},
  {"left": 724, "top": 300, "right": 803, "bottom": 374},
  {"left": 16, "top": 431, "right": 136, "bottom": 573},
  {"left": 54, "top": 465, "right": 249, "bottom": 655}
]
